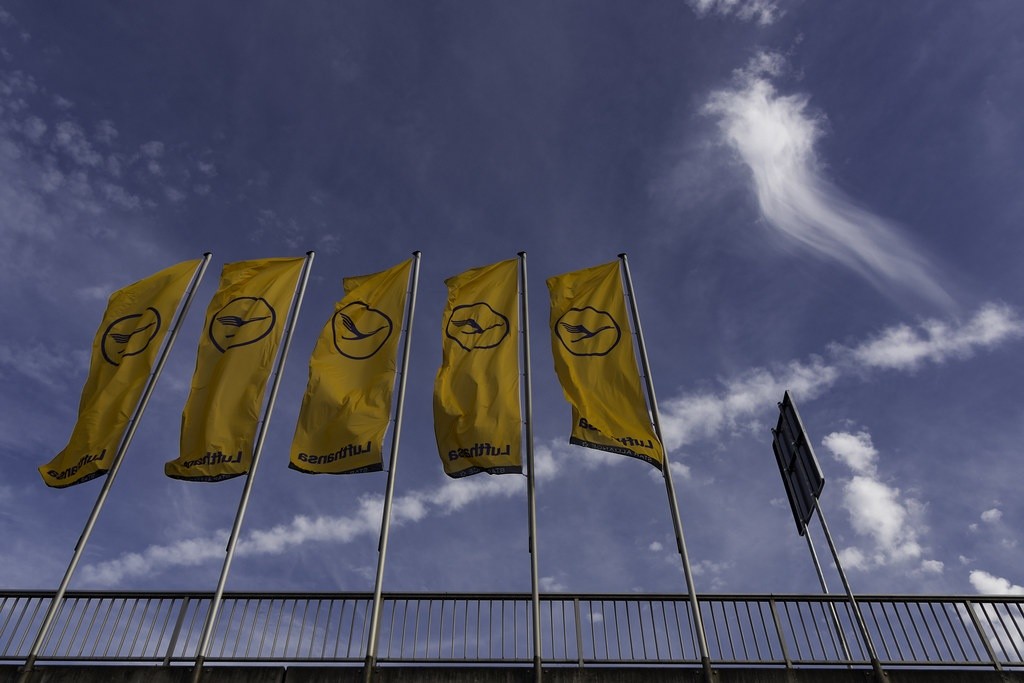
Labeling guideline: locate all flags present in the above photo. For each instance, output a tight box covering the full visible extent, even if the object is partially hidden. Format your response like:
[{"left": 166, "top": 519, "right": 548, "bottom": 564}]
[
  {"left": 433, "top": 258, "right": 523, "bottom": 479},
  {"left": 38, "top": 258, "right": 202, "bottom": 489},
  {"left": 164, "top": 256, "right": 306, "bottom": 483},
  {"left": 289, "top": 257, "right": 413, "bottom": 474},
  {"left": 546, "top": 260, "right": 663, "bottom": 473}
]
